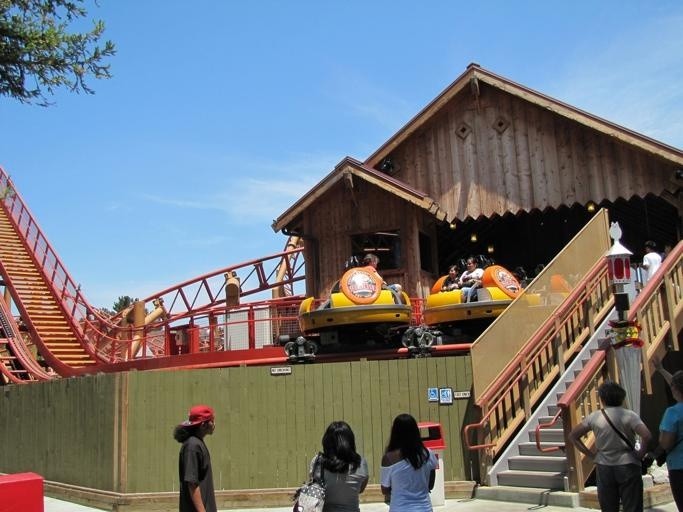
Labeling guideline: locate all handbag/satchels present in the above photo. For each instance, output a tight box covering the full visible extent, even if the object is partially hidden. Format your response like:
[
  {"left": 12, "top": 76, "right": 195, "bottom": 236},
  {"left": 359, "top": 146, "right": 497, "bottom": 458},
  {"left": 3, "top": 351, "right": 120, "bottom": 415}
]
[
  {"left": 655, "top": 446, "right": 667, "bottom": 467},
  {"left": 642, "top": 453, "right": 654, "bottom": 474}
]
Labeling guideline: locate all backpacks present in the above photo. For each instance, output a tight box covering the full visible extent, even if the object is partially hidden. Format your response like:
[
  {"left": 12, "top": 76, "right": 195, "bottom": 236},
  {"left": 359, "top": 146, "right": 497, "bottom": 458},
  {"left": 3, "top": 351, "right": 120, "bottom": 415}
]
[{"left": 293, "top": 451, "right": 326, "bottom": 512}]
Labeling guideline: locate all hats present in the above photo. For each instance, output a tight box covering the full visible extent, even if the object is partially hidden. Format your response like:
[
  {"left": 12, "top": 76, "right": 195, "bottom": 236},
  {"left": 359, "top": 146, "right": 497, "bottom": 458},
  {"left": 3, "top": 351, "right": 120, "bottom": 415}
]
[{"left": 181, "top": 406, "right": 214, "bottom": 427}]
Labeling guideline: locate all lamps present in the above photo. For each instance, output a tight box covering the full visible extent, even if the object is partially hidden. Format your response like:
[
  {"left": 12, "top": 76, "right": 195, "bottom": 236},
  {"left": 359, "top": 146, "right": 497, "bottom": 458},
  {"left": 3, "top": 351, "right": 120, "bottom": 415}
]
[
  {"left": 471, "top": 231, "right": 477, "bottom": 243},
  {"left": 450, "top": 219, "right": 457, "bottom": 229},
  {"left": 587, "top": 201, "right": 596, "bottom": 213}
]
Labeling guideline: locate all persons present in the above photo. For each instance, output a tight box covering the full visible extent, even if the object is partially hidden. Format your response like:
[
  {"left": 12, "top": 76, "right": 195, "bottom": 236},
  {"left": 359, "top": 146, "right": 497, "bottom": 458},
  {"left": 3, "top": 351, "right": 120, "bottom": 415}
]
[
  {"left": 363, "top": 254, "right": 402, "bottom": 304},
  {"left": 457, "top": 256, "right": 484, "bottom": 303},
  {"left": 641, "top": 240, "right": 661, "bottom": 286},
  {"left": 435, "top": 263, "right": 462, "bottom": 294},
  {"left": 379, "top": 412, "right": 436, "bottom": 512},
  {"left": 654, "top": 372, "right": 682, "bottom": 511},
  {"left": 172, "top": 405, "right": 218, "bottom": 512},
  {"left": 566, "top": 381, "right": 652, "bottom": 512},
  {"left": 291, "top": 420, "right": 368, "bottom": 511}
]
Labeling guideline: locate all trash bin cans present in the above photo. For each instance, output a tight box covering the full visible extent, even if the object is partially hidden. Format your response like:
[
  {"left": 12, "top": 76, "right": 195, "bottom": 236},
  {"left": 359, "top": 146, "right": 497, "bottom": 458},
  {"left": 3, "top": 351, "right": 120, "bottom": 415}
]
[{"left": 416, "top": 422, "right": 447, "bottom": 507}]
